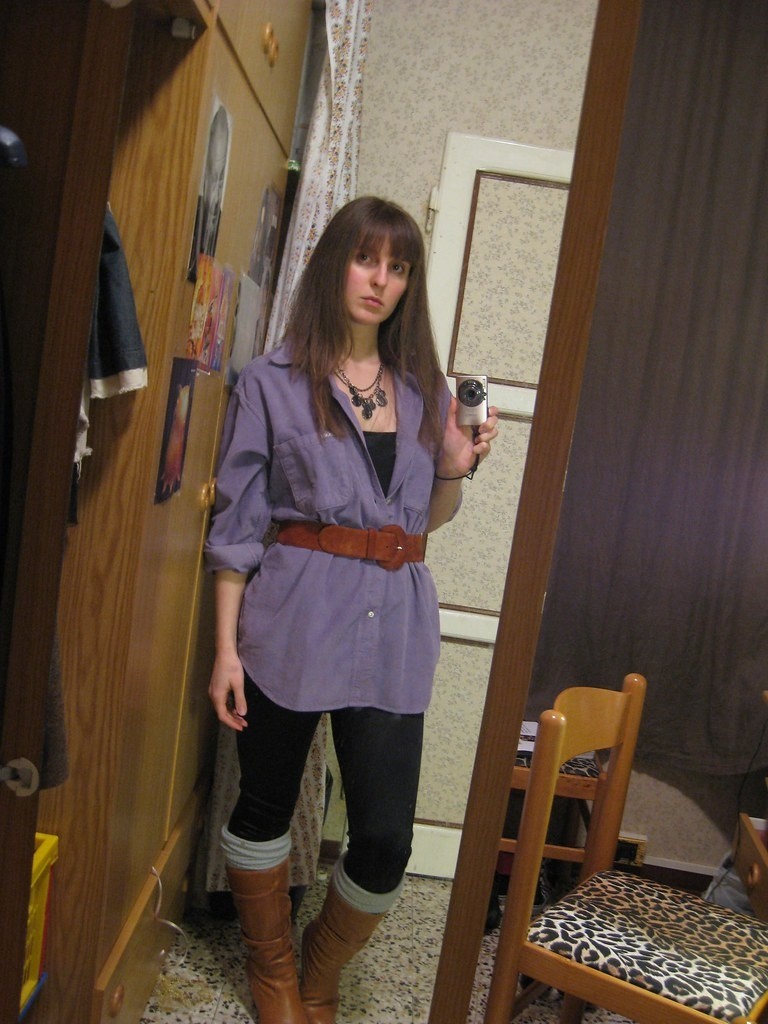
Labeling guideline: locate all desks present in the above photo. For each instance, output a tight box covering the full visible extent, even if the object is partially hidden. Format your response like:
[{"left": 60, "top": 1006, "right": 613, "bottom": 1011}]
[{"left": 499, "top": 750, "right": 608, "bottom": 862}]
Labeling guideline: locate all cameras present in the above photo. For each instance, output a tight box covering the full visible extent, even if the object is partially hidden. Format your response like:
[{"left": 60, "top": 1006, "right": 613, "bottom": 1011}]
[{"left": 457, "top": 375, "right": 489, "bottom": 426}]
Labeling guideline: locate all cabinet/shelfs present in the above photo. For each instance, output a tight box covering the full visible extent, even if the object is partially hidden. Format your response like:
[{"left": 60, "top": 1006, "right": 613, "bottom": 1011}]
[{"left": 36, "top": 0, "right": 314, "bottom": 1024}]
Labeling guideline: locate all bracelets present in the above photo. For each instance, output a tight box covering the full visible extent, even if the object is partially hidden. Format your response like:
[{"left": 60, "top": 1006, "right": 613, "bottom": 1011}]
[{"left": 435, "top": 472, "right": 475, "bottom": 481}]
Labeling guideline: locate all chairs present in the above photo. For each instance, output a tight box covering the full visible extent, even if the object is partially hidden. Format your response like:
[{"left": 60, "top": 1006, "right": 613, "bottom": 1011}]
[{"left": 482, "top": 673, "right": 768, "bottom": 1024}]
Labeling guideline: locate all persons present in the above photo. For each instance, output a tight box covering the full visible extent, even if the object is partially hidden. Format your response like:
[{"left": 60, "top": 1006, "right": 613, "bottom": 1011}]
[{"left": 201, "top": 196, "right": 497, "bottom": 1024}]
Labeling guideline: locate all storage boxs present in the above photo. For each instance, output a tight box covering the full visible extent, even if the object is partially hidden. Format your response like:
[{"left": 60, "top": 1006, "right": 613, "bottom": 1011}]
[
  {"left": 17, "top": 972, "right": 48, "bottom": 1024},
  {"left": 19, "top": 832, "right": 60, "bottom": 1012}
]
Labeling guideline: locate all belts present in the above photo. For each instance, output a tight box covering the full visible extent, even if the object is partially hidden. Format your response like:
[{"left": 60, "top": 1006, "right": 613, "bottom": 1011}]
[{"left": 277, "top": 522, "right": 428, "bottom": 570}]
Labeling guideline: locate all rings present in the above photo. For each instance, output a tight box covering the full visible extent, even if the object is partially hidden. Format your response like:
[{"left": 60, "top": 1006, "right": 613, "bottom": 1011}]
[{"left": 210, "top": 703, "right": 216, "bottom": 711}]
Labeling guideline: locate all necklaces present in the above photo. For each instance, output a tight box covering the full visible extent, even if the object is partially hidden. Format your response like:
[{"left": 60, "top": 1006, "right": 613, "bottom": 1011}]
[{"left": 332, "top": 359, "right": 387, "bottom": 421}]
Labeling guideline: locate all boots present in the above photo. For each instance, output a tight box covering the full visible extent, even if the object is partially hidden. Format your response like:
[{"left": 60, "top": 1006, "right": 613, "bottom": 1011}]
[
  {"left": 299, "top": 875, "right": 389, "bottom": 1024},
  {"left": 227, "top": 856, "right": 310, "bottom": 1024}
]
